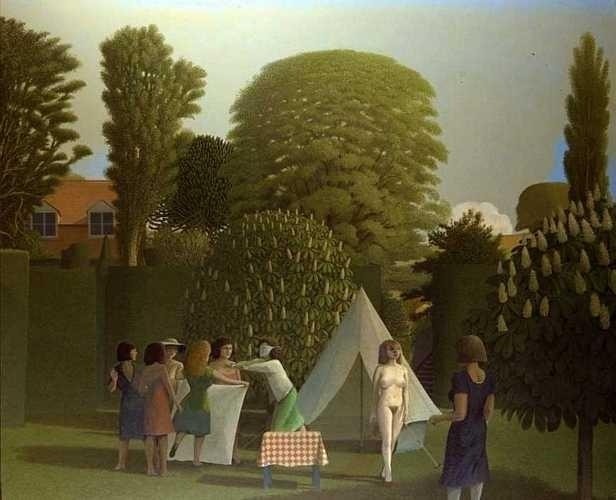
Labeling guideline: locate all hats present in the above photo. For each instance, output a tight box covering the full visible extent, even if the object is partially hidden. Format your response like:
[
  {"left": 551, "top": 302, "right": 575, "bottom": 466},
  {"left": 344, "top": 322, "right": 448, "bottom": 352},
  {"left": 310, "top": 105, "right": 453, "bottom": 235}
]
[{"left": 160, "top": 338, "right": 185, "bottom": 352}]
